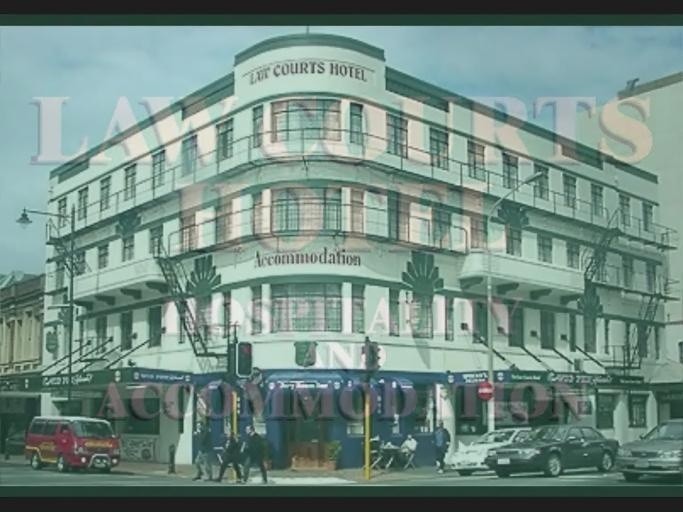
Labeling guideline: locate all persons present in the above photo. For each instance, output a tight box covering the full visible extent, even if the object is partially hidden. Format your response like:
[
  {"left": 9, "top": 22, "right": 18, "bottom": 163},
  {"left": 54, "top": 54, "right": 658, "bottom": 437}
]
[
  {"left": 241, "top": 425, "right": 268, "bottom": 484},
  {"left": 397, "top": 433, "right": 417, "bottom": 470},
  {"left": 192, "top": 421, "right": 214, "bottom": 482},
  {"left": 212, "top": 429, "right": 242, "bottom": 483},
  {"left": 431, "top": 419, "right": 450, "bottom": 472}
]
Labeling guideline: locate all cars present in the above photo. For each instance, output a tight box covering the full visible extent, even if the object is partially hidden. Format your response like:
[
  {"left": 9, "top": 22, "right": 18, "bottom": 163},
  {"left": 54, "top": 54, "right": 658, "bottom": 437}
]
[
  {"left": 619, "top": 418, "right": 682, "bottom": 481},
  {"left": 485, "top": 424, "right": 619, "bottom": 476},
  {"left": 449, "top": 426, "right": 534, "bottom": 476}
]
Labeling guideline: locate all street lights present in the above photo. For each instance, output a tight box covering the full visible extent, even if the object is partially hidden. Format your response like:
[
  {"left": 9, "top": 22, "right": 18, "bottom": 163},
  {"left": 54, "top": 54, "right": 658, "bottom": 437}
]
[
  {"left": 484, "top": 171, "right": 544, "bottom": 431},
  {"left": 15, "top": 204, "right": 74, "bottom": 415}
]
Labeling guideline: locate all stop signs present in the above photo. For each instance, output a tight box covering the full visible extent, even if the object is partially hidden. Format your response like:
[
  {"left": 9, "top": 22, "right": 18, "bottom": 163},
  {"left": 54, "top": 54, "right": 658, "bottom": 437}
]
[{"left": 476, "top": 381, "right": 493, "bottom": 401}]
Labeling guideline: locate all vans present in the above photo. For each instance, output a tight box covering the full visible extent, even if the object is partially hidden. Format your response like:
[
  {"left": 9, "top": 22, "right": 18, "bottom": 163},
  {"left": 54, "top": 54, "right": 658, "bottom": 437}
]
[{"left": 23, "top": 415, "right": 121, "bottom": 473}]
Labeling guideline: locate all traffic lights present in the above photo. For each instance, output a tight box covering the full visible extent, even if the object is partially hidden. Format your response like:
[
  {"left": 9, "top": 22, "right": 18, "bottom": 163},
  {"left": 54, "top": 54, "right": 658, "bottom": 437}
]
[{"left": 235, "top": 341, "right": 253, "bottom": 379}]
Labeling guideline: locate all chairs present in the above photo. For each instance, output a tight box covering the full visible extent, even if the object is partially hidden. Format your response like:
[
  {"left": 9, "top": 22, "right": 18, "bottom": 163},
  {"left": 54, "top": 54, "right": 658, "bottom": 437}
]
[{"left": 361, "top": 441, "right": 419, "bottom": 470}]
